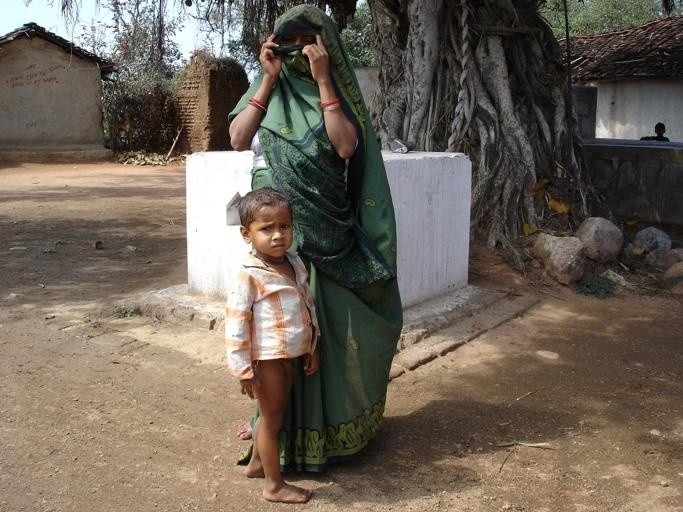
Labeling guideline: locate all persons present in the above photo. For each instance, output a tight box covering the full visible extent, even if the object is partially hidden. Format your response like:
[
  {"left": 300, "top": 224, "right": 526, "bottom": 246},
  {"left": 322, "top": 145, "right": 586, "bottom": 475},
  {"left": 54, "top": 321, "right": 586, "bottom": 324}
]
[
  {"left": 640, "top": 123, "right": 670, "bottom": 142},
  {"left": 225, "top": 188, "right": 320, "bottom": 503},
  {"left": 228, "top": 3, "right": 402, "bottom": 476}
]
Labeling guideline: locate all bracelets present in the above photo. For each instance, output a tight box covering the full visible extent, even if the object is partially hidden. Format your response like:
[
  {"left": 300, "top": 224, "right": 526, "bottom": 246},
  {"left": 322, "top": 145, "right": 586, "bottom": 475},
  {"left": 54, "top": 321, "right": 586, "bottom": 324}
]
[
  {"left": 248, "top": 97, "right": 266, "bottom": 112},
  {"left": 321, "top": 98, "right": 340, "bottom": 110}
]
